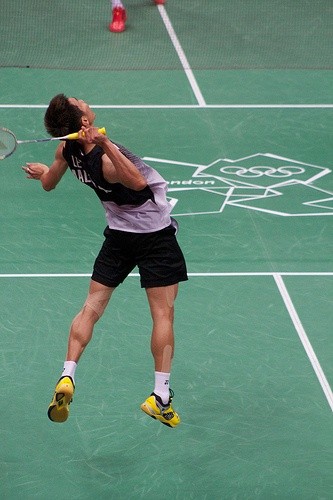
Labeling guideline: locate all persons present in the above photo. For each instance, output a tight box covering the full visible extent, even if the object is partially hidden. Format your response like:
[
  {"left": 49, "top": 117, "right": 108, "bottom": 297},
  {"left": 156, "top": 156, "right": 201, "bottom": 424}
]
[
  {"left": 109, "top": 1, "right": 167, "bottom": 31},
  {"left": 22, "top": 95, "right": 187, "bottom": 429}
]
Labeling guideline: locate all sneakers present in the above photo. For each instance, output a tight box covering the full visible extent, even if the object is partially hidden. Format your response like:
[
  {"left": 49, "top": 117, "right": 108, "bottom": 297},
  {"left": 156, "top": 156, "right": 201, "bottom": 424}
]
[
  {"left": 140, "top": 388, "right": 180, "bottom": 428},
  {"left": 154, "top": 0, "right": 165, "bottom": 4},
  {"left": 47, "top": 377, "right": 75, "bottom": 423},
  {"left": 109, "top": 7, "right": 126, "bottom": 32}
]
